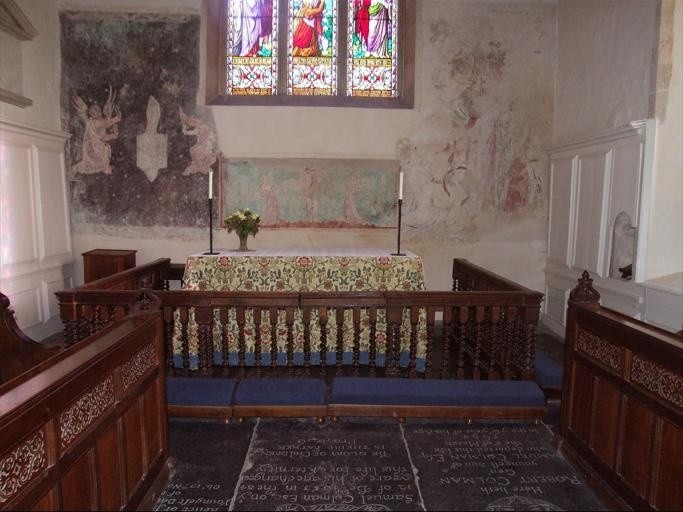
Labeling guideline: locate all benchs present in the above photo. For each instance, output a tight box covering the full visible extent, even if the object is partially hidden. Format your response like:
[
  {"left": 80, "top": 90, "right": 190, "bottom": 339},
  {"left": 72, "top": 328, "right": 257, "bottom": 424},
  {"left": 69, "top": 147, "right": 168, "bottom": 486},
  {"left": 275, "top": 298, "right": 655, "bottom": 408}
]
[{"left": 164, "top": 374, "right": 550, "bottom": 426}]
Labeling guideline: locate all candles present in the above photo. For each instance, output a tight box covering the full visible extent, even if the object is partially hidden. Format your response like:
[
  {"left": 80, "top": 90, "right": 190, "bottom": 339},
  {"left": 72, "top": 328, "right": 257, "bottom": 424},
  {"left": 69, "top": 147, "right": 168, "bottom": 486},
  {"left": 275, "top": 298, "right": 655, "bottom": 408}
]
[
  {"left": 399, "top": 170, "right": 404, "bottom": 198},
  {"left": 208, "top": 165, "right": 213, "bottom": 199}
]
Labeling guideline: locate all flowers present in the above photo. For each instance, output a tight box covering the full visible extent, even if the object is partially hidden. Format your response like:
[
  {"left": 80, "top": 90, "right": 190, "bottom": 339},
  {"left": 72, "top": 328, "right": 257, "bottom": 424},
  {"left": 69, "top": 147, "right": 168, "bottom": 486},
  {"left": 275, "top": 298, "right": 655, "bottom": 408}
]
[{"left": 222, "top": 207, "right": 261, "bottom": 237}]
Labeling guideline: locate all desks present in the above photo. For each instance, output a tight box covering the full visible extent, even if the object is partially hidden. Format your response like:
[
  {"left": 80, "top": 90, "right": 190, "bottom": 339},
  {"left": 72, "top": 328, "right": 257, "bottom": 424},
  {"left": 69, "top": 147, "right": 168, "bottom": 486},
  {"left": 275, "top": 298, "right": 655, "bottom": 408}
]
[{"left": 170, "top": 248, "right": 429, "bottom": 375}]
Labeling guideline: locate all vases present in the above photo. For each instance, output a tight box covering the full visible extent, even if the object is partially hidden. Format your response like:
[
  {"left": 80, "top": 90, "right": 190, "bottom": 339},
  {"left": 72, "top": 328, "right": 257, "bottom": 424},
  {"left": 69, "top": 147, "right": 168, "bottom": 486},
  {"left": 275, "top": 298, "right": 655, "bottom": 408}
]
[{"left": 233, "top": 231, "right": 253, "bottom": 251}]
